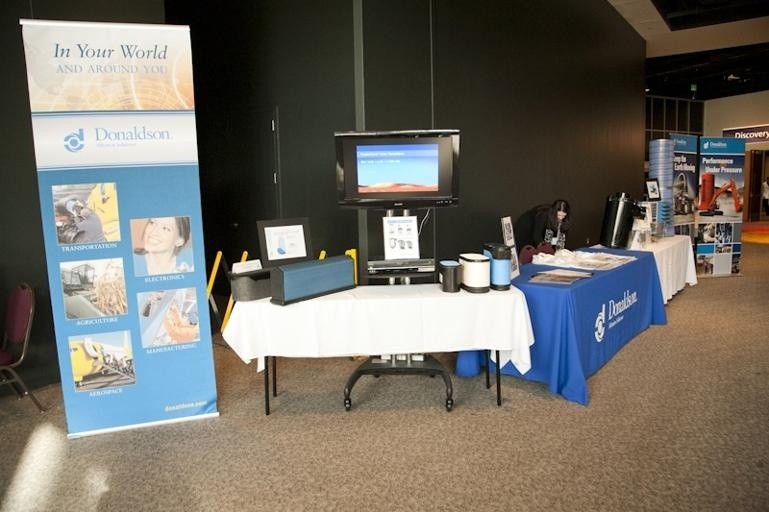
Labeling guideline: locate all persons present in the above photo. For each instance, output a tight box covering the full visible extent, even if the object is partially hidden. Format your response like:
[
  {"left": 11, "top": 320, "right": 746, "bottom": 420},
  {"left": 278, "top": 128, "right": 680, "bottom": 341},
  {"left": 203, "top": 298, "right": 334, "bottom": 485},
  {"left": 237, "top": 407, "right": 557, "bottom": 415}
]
[
  {"left": 132, "top": 217, "right": 194, "bottom": 276},
  {"left": 73, "top": 207, "right": 107, "bottom": 243},
  {"left": 514, "top": 199, "right": 571, "bottom": 259},
  {"left": 760, "top": 177, "right": 769, "bottom": 216},
  {"left": 703, "top": 223, "right": 716, "bottom": 244}
]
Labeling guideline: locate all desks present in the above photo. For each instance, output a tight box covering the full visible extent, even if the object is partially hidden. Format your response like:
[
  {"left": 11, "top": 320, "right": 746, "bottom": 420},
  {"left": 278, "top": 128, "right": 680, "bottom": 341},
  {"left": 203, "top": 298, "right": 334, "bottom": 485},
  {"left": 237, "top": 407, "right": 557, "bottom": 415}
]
[
  {"left": 220, "top": 249, "right": 535, "bottom": 414},
  {"left": 454, "top": 235, "right": 699, "bottom": 407}
]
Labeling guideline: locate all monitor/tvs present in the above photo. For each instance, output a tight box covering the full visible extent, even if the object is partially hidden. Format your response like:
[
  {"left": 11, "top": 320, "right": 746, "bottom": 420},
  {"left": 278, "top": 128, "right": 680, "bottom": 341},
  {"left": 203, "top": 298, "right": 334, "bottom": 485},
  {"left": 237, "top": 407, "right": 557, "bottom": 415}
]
[{"left": 335, "top": 129, "right": 461, "bottom": 209}]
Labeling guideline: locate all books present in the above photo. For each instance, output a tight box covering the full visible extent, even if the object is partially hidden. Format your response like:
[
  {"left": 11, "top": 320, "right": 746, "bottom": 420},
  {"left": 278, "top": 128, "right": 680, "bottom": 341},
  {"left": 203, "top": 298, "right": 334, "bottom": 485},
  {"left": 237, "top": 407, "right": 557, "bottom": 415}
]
[{"left": 527, "top": 268, "right": 593, "bottom": 285}]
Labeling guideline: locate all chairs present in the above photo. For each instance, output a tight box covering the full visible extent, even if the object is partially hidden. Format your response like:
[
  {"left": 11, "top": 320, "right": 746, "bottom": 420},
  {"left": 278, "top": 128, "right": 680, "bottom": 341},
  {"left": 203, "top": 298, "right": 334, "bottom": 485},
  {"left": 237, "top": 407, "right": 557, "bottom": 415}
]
[{"left": 1, "top": 281, "right": 46, "bottom": 414}]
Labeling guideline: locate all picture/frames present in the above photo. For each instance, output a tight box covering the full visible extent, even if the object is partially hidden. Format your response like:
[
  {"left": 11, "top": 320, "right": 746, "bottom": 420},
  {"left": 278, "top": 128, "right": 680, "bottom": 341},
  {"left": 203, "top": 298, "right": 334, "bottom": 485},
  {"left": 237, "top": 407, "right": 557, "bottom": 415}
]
[
  {"left": 644, "top": 178, "right": 662, "bottom": 202},
  {"left": 255, "top": 218, "right": 314, "bottom": 268}
]
